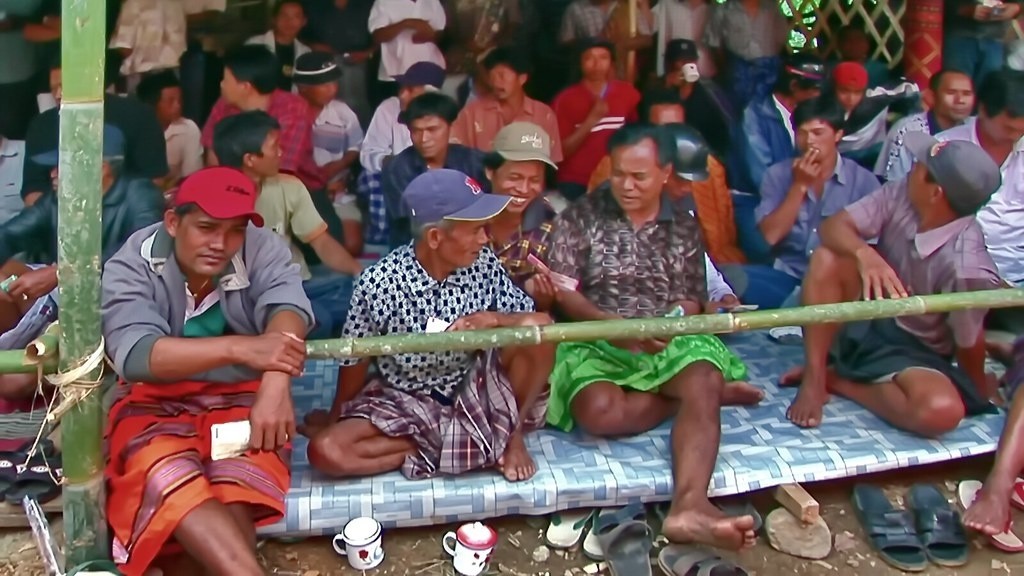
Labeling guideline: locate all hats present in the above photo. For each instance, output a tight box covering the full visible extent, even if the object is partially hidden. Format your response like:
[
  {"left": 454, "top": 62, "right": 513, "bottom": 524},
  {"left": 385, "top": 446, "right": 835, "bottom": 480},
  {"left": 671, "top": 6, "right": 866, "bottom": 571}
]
[
  {"left": 495, "top": 120, "right": 560, "bottom": 170},
  {"left": 29, "top": 122, "right": 126, "bottom": 165},
  {"left": 833, "top": 63, "right": 868, "bottom": 91},
  {"left": 293, "top": 50, "right": 343, "bottom": 85},
  {"left": 174, "top": 166, "right": 265, "bottom": 230},
  {"left": 903, "top": 130, "right": 1002, "bottom": 205},
  {"left": 390, "top": 60, "right": 448, "bottom": 91},
  {"left": 397, "top": 93, "right": 459, "bottom": 124},
  {"left": 663, "top": 39, "right": 701, "bottom": 60},
  {"left": 400, "top": 167, "right": 515, "bottom": 220}
]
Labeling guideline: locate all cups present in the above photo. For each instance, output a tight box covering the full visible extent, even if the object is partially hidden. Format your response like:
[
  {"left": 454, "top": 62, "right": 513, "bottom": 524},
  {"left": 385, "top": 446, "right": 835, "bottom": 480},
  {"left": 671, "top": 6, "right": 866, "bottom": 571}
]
[
  {"left": 333, "top": 518, "right": 385, "bottom": 570},
  {"left": 443, "top": 522, "right": 496, "bottom": 575},
  {"left": 677, "top": 64, "right": 700, "bottom": 83}
]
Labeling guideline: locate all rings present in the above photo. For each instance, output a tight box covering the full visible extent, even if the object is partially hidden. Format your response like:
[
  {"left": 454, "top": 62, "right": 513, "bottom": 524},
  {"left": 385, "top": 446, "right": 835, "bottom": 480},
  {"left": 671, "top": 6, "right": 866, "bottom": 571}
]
[{"left": 22, "top": 292, "right": 28, "bottom": 300}]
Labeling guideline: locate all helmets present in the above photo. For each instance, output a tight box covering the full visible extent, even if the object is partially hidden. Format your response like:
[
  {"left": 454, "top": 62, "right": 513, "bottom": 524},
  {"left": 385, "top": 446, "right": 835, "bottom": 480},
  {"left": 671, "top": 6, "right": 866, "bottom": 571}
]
[
  {"left": 783, "top": 51, "right": 827, "bottom": 83},
  {"left": 661, "top": 121, "right": 711, "bottom": 182}
]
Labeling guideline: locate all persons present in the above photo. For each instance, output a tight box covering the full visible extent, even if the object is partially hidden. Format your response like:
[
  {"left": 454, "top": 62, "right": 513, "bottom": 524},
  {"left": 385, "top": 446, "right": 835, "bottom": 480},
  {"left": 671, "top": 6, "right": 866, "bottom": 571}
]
[{"left": 0, "top": 0, "right": 1024, "bottom": 576}]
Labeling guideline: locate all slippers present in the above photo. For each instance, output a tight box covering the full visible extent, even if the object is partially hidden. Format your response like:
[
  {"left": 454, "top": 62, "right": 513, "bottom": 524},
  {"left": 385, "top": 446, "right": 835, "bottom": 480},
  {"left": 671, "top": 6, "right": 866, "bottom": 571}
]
[
  {"left": 594, "top": 499, "right": 750, "bottom": 576},
  {"left": 0, "top": 437, "right": 62, "bottom": 505},
  {"left": 545, "top": 504, "right": 624, "bottom": 560},
  {"left": 960, "top": 476, "right": 1024, "bottom": 552},
  {"left": 850, "top": 480, "right": 968, "bottom": 572}
]
